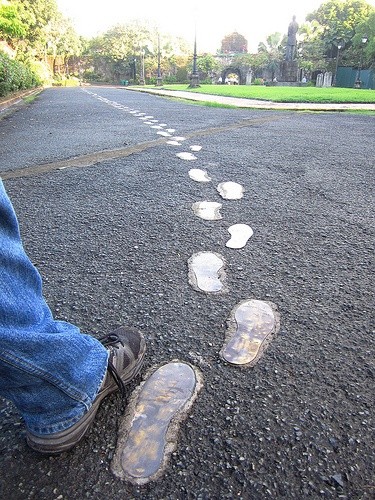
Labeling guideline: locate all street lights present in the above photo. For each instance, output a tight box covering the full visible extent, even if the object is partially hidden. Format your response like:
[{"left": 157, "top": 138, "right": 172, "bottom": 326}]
[
  {"left": 354, "top": 37, "right": 368, "bottom": 90},
  {"left": 331, "top": 44, "right": 343, "bottom": 88}
]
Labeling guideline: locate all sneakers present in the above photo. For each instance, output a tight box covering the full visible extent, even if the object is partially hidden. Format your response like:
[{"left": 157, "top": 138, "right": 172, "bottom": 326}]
[{"left": 27, "top": 326, "right": 147, "bottom": 454}]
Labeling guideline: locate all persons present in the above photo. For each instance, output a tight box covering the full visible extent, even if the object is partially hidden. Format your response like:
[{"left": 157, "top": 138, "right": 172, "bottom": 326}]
[{"left": 0, "top": 176, "right": 146, "bottom": 455}]
